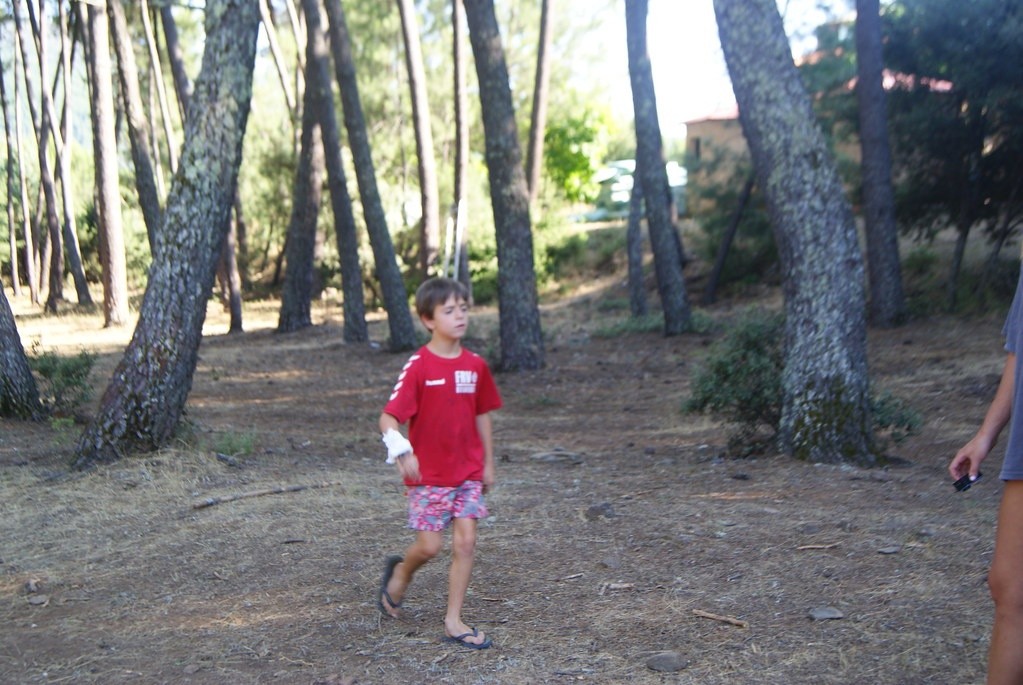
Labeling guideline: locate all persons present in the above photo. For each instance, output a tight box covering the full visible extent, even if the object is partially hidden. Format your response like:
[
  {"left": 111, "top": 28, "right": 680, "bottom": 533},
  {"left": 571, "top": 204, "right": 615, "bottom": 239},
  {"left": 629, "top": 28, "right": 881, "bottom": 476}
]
[
  {"left": 948, "top": 261, "right": 1023, "bottom": 685},
  {"left": 378, "top": 277, "right": 504, "bottom": 650}
]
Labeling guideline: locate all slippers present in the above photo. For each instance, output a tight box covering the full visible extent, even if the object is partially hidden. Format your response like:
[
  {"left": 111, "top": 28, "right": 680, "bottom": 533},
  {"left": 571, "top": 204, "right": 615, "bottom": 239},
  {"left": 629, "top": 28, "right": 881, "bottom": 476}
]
[
  {"left": 377, "top": 555, "right": 403, "bottom": 616},
  {"left": 442, "top": 627, "right": 492, "bottom": 649}
]
